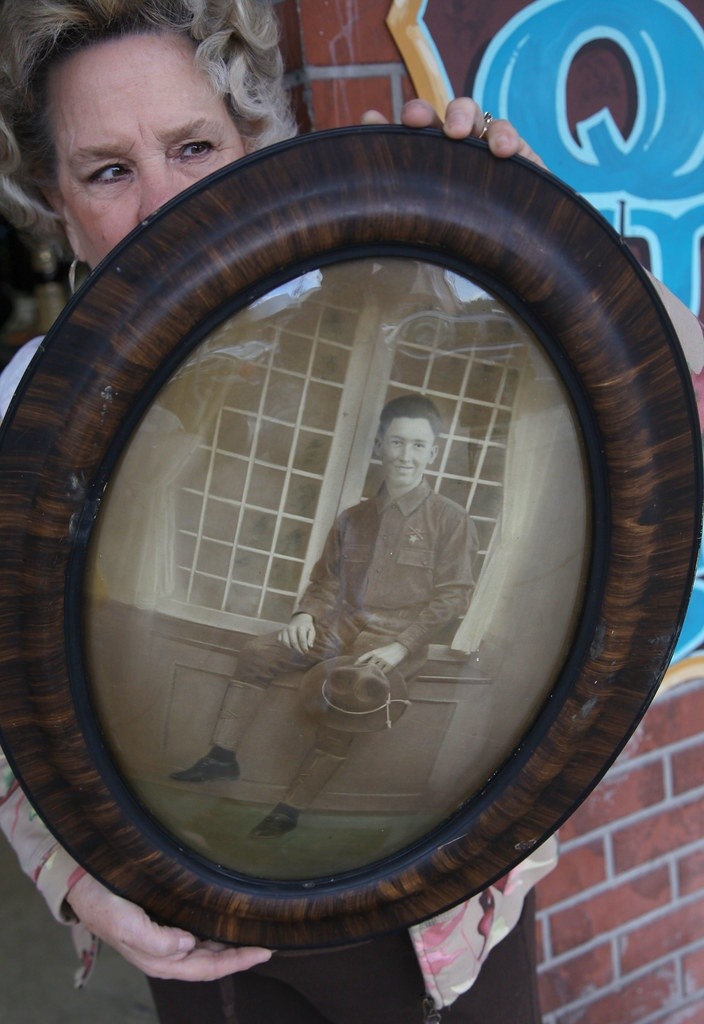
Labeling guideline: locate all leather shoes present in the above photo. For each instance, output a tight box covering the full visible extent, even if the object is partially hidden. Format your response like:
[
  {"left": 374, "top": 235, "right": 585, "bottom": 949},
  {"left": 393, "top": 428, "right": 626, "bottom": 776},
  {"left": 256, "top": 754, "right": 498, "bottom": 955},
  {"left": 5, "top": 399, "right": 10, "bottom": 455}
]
[
  {"left": 251, "top": 812, "right": 299, "bottom": 839},
  {"left": 166, "top": 755, "right": 240, "bottom": 781}
]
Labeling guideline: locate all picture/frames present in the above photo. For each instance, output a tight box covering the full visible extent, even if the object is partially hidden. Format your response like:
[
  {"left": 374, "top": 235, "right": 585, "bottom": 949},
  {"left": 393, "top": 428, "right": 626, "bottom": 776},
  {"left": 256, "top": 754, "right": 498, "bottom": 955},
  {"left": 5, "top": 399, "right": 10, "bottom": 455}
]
[{"left": 0, "top": 124, "right": 702, "bottom": 942}]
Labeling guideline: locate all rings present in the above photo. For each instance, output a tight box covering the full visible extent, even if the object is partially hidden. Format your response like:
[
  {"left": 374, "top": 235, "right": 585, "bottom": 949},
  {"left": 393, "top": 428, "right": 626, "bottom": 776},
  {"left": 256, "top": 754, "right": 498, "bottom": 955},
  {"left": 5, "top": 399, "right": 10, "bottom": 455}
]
[{"left": 477, "top": 112, "right": 494, "bottom": 139}]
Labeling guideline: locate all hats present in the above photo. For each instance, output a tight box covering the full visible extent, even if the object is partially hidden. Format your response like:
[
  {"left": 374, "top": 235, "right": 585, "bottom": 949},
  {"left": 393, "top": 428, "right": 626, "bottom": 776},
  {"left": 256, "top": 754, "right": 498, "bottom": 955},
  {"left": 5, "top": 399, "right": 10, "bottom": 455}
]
[{"left": 297, "top": 655, "right": 410, "bottom": 732}]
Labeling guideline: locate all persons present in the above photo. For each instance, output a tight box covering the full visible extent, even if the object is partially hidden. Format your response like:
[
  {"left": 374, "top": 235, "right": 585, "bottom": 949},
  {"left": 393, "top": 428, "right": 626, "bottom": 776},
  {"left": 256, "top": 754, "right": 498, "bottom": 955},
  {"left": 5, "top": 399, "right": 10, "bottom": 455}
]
[
  {"left": 0, "top": 0, "right": 704, "bottom": 1024},
  {"left": 170, "top": 395, "right": 480, "bottom": 843}
]
